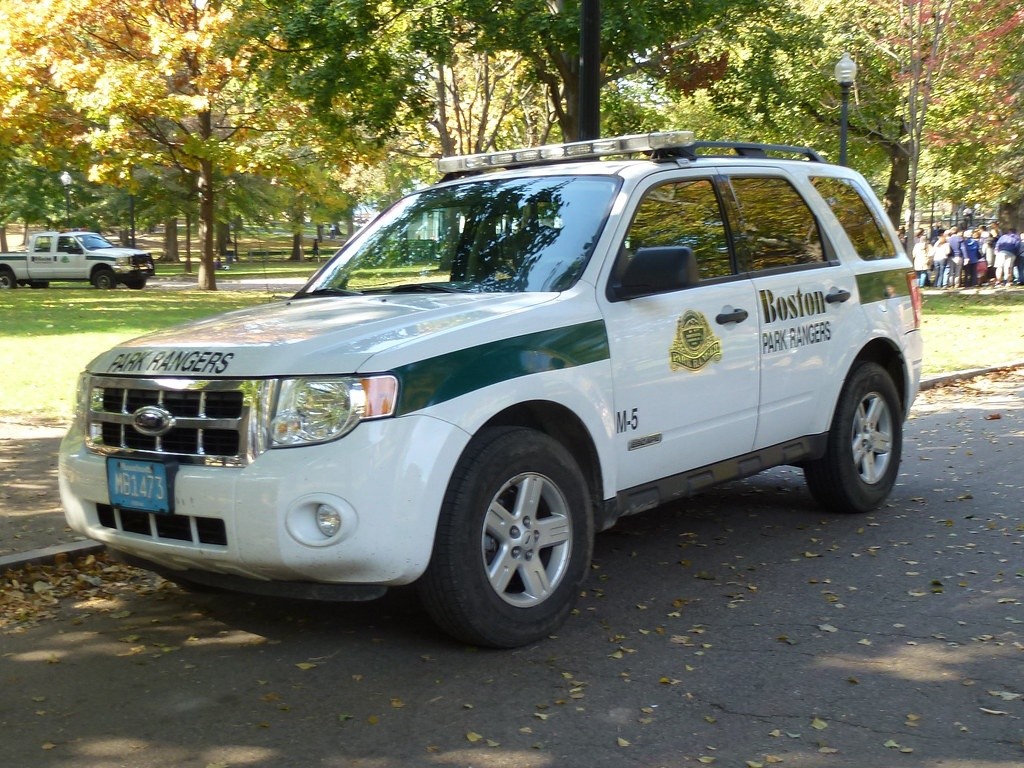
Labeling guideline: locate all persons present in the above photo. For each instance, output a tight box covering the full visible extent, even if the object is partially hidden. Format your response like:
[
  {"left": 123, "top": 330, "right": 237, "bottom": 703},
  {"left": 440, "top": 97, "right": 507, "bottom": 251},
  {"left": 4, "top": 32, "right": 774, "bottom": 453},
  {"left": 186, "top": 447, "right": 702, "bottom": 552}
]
[
  {"left": 214, "top": 257, "right": 227, "bottom": 271},
  {"left": 311, "top": 239, "right": 319, "bottom": 259},
  {"left": 896, "top": 218, "right": 1024, "bottom": 291},
  {"left": 329, "top": 224, "right": 338, "bottom": 239}
]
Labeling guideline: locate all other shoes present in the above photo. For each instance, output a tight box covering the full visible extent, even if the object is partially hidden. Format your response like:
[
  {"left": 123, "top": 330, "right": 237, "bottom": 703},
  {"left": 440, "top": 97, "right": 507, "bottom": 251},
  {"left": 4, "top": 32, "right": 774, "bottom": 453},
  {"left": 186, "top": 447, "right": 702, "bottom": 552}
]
[
  {"left": 993, "top": 282, "right": 1001, "bottom": 288},
  {"left": 1005, "top": 282, "right": 1014, "bottom": 286},
  {"left": 942, "top": 285, "right": 948, "bottom": 290},
  {"left": 957, "top": 286, "right": 966, "bottom": 290}
]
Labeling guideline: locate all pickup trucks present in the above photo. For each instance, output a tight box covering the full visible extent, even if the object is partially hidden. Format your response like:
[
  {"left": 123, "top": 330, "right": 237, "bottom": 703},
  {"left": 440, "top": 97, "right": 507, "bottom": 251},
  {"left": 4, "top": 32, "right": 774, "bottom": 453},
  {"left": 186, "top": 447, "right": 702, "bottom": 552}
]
[{"left": 0, "top": 230, "right": 155, "bottom": 290}]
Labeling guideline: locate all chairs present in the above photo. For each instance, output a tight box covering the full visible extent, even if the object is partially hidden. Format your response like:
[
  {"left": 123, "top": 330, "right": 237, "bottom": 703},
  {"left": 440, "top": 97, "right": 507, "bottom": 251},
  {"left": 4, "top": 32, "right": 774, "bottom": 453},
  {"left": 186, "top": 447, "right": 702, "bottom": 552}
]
[{"left": 619, "top": 246, "right": 696, "bottom": 295}]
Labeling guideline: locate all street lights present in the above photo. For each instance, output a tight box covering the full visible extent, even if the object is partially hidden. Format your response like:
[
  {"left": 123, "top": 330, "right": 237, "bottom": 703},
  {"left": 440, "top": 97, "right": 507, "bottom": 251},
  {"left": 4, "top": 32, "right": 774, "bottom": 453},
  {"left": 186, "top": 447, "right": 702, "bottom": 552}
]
[{"left": 829, "top": 51, "right": 857, "bottom": 165}]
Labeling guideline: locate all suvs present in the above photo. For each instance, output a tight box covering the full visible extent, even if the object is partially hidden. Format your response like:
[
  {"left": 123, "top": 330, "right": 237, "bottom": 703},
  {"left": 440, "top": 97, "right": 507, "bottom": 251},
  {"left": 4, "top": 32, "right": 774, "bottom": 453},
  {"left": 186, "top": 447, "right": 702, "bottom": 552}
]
[{"left": 56, "top": 132, "right": 927, "bottom": 652}]
